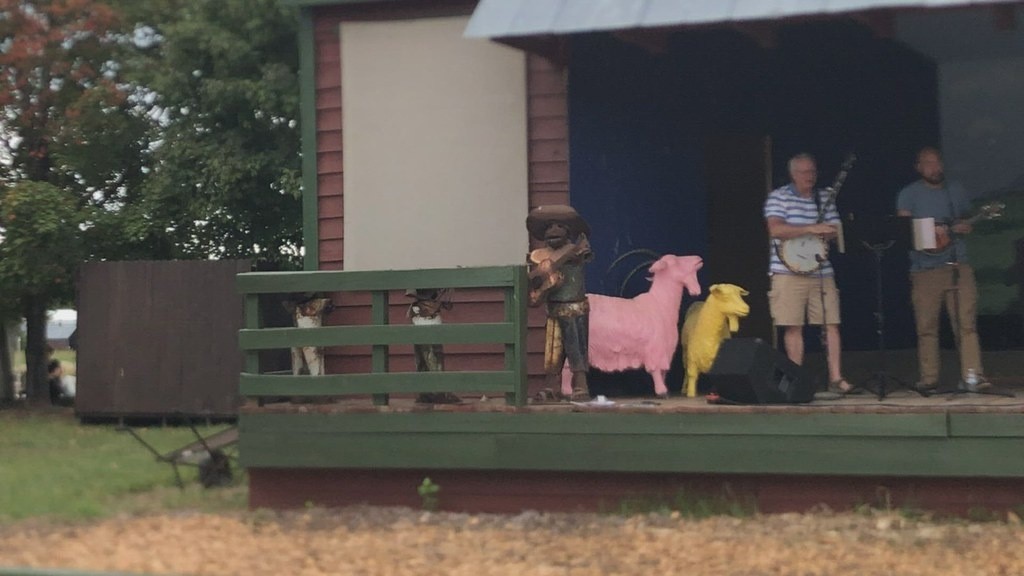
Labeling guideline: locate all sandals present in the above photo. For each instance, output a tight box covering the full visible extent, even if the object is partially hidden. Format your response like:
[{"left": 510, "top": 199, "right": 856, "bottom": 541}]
[{"left": 826, "top": 377, "right": 863, "bottom": 394}]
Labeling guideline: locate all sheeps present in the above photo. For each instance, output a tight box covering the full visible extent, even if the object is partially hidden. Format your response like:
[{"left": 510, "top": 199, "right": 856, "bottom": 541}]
[
  {"left": 559, "top": 255, "right": 704, "bottom": 397},
  {"left": 680, "top": 283, "right": 751, "bottom": 398}
]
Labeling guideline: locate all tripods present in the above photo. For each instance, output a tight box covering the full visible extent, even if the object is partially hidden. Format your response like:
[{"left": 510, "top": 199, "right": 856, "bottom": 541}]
[{"left": 839, "top": 215, "right": 1015, "bottom": 404}]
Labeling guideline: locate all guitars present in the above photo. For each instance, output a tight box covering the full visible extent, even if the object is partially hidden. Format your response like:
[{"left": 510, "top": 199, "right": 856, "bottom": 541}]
[
  {"left": 778, "top": 153, "right": 859, "bottom": 276},
  {"left": 920, "top": 199, "right": 1006, "bottom": 256}
]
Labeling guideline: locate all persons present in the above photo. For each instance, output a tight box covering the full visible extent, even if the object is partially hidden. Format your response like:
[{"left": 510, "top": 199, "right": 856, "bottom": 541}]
[
  {"left": 283, "top": 291, "right": 333, "bottom": 404},
  {"left": 404, "top": 287, "right": 461, "bottom": 403},
  {"left": 45, "top": 344, "right": 62, "bottom": 396},
  {"left": 897, "top": 147, "right": 991, "bottom": 393},
  {"left": 764, "top": 153, "right": 864, "bottom": 395},
  {"left": 526, "top": 205, "right": 595, "bottom": 402}
]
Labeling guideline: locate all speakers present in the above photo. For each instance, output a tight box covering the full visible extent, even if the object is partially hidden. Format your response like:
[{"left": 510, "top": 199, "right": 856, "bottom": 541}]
[{"left": 703, "top": 337, "right": 818, "bottom": 405}]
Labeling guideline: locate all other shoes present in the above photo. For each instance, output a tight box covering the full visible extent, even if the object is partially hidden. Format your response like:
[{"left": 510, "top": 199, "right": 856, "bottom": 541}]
[
  {"left": 916, "top": 384, "right": 937, "bottom": 394},
  {"left": 963, "top": 376, "right": 994, "bottom": 391}
]
